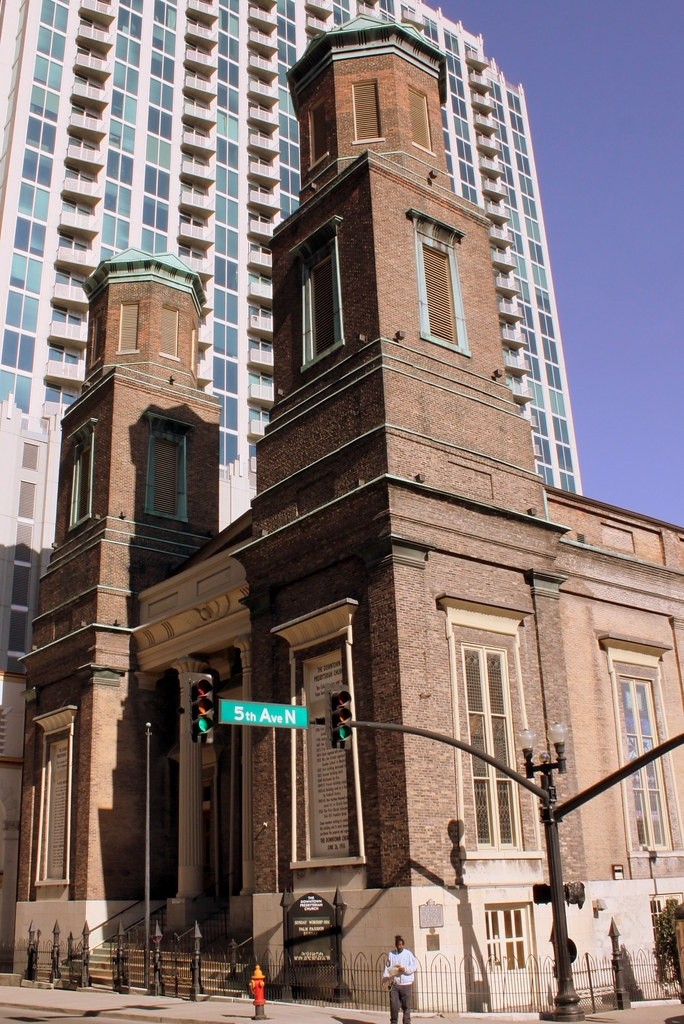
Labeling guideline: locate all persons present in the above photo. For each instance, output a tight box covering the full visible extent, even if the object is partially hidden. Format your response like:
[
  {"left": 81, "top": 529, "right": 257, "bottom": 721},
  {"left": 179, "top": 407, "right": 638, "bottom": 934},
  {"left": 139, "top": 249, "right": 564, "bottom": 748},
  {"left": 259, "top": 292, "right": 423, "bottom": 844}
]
[{"left": 383, "top": 936, "right": 416, "bottom": 1024}]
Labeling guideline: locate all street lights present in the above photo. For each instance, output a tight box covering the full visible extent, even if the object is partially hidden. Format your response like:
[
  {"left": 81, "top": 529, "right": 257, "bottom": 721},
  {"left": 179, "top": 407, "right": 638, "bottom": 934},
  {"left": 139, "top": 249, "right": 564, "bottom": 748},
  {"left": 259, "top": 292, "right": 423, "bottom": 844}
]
[{"left": 517, "top": 718, "right": 594, "bottom": 1024}]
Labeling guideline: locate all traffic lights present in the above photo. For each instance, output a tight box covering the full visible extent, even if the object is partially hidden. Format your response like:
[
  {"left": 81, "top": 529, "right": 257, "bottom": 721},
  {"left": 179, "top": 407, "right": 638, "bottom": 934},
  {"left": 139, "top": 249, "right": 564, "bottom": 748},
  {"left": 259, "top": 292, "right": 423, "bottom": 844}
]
[
  {"left": 185, "top": 676, "right": 218, "bottom": 746},
  {"left": 324, "top": 684, "right": 351, "bottom": 750}
]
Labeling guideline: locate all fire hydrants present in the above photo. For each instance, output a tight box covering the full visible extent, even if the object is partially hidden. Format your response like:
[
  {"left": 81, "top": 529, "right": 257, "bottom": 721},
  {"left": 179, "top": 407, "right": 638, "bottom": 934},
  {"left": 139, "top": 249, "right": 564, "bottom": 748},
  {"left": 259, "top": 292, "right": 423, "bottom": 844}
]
[{"left": 250, "top": 962, "right": 267, "bottom": 1021}]
